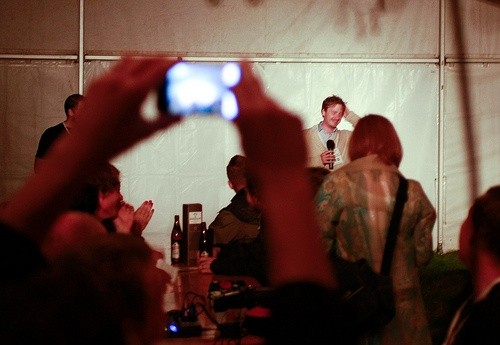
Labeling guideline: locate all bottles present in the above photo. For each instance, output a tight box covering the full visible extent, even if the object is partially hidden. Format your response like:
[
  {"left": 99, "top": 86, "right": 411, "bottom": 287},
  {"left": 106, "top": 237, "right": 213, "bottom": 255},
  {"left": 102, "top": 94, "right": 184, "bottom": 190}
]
[
  {"left": 171, "top": 215, "right": 184, "bottom": 263},
  {"left": 198, "top": 222, "right": 210, "bottom": 257}
]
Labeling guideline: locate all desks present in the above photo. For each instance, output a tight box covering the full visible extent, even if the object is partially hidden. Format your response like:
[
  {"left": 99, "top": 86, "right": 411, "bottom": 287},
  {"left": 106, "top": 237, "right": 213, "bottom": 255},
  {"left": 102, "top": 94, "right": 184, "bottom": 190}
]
[{"left": 123, "top": 257, "right": 271, "bottom": 345}]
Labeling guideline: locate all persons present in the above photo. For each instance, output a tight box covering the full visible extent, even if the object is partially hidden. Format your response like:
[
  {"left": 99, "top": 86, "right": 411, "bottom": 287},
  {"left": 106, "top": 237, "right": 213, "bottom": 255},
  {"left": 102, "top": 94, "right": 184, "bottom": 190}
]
[
  {"left": 308, "top": 112, "right": 436, "bottom": 345},
  {"left": 429, "top": 183, "right": 500, "bottom": 345},
  {"left": 0, "top": 55, "right": 357, "bottom": 345},
  {"left": 302, "top": 96, "right": 363, "bottom": 173}
]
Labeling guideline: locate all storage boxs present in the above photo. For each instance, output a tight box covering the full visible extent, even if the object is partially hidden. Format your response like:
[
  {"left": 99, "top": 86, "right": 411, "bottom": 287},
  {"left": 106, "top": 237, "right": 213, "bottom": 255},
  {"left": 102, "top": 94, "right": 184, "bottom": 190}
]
[{"left": 183, "top": 203, "right": 202, "bottom": 267}]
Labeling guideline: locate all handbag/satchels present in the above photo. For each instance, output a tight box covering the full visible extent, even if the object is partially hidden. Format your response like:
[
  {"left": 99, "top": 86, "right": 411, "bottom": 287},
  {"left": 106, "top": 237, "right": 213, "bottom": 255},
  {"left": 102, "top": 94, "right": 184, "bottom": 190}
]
[{"left": 328, "top": 249, "right": 395, "bottom": 331}]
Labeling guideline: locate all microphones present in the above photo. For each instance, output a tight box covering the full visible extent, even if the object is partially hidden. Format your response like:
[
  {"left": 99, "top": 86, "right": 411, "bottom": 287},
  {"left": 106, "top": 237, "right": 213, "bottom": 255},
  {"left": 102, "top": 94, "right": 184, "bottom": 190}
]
[{"left": 327, "top": 139, "right": 335, "bottom": 169}]
